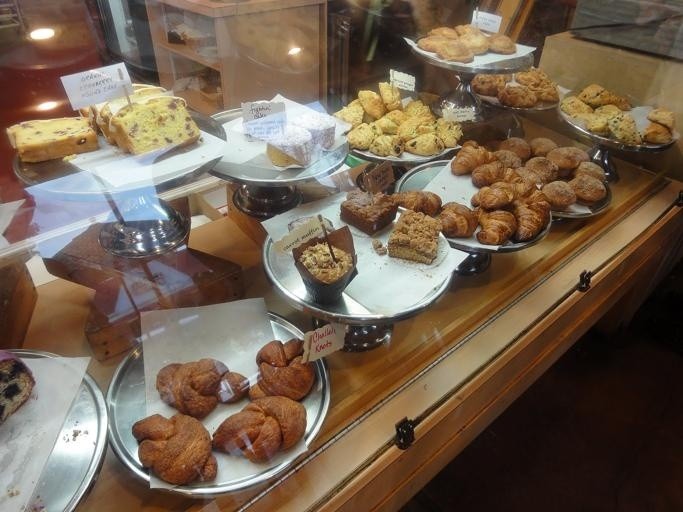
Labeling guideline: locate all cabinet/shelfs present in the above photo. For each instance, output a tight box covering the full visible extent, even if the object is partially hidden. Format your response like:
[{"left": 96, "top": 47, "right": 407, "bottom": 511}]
[
  {"left": 0, "top": 0, "right": 683, "bottom": 512},
  {"left": 143, "top": 0, "right": 328, "bottom": 118}
]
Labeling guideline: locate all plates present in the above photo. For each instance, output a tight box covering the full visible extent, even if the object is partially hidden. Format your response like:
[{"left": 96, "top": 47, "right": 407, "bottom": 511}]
[
  {"left": 201, "top": 102, "right": 351, "bottom": 187},
  {"left": 108, "top": 306, "right": 332, "bottom": 494},
  {"left": 261, "top": 192, "right": 457, "bottom": 326},
  {"left": 557, "top": 95, "right": 680, "bottom": 150},
  {"left": 394, "top": 160, "right": 553, "bottom": 253},
  {"left": 536, "top": 166, "right": 615, "bottom": 222},
  {"left": 2, "top": 344, "right": 110, "bottom": 511},
  {"left": 8, "top": 97, "right": 230, "bottom": 193},
  {"left": 467, "top": 70, "right": 571, "bottom": 113},
  {"left": 403, "top": 21, "right": 536, "bottom": 75},
  {"left": 344, "top": 126, "right": 464, "bottom": 165}
]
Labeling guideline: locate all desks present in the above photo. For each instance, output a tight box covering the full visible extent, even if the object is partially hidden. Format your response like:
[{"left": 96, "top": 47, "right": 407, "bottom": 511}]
[{"left": 520, "top": 31, "right": 683, "bottom": 182}]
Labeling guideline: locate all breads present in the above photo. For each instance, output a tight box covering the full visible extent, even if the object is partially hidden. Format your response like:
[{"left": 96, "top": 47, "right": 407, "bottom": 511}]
[
  {"left": 1, "top": 348, "right": 35, "bottom": 424},
  {"left": 332, "top": 81, "right": 464, "bottom": 156},
  {"left": 472, "top": 64, "right": 558, "bottom": 108},
  {"left": 4, "top": 79, "right": 201, "bottom": 165},
  {"left": 263, "top": 111, "right": 335, "bottom": 166},
  {"left": 488, "top": 136, "right": 608, "bottom": 209},
  {"left": 281, "top": 187, "right": 444, "bottom": 303},
  {"left": 386, "top": 138, "right": 551, "bottom": 245},
  {"left": 559, "top": 82, "right": 675, "bottom": 147},
  {"left": 129, "top": 337, "right": 320, "bottom": 485},
  {"left": 416, "top": 23, "right": 518, "bottom": 64}
]
